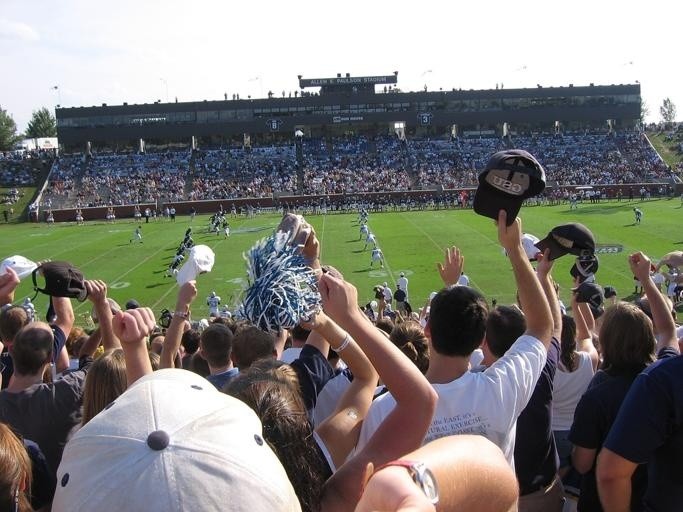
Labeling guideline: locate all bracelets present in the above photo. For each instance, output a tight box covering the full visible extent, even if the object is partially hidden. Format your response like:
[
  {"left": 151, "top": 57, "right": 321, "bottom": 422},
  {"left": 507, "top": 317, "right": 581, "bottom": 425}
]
[
  {"left": 171, "top": 309, "right": 192, "bottom": 321},
  {"left": 330, "top": 332, "right": 351, "bottom": 354}
]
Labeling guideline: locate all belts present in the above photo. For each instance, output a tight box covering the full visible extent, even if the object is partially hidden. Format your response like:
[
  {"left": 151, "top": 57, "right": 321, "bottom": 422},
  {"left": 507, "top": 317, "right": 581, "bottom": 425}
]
[{"left": 519, "top": 474, "right": 555, "bottom": 497}]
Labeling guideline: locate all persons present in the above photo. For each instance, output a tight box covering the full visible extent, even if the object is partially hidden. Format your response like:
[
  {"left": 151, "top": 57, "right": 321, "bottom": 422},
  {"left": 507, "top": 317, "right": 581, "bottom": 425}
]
[
  {"left": 0, "top": 421, "right": 35, "bottom": 512},
  {"left": 373, "top": 245, "right": 465, "bottom": 400},
  {"left": 208, "top": 225, "right": 230, "bottom": 239},
  {"left": 158, "top": 280, "right": 197, "bottom": 369},
  {"left": 481, "top": 248, "right": 565, "bottom": 512},
  {"left": 206, "top": 291, "right": 220, "bottom": 313},
  {"left": 151, "top": 335, "right": 168, "bottom": 355},
  {"left": 222, "top": 273, "right": 440, "bottom": 512},
  {"left": 363, "top": 232, "right": 376, "bottom": 250},
  {"left": 381, "top": 282, "right": 393, "bottom": 305},
  {"left": 179, "top": 328, "right": 201, "bottom": 369},
  {"left": 19, "top": 436, "right": 56, "bottom": 511},
  {"left": 353, "top": 210, "right": 556, "bottom": 478},
  {"left": 370, "top": 247, "right": 384, "bottom": 268},
  {"left": 1, "top": 83, "right": 683, "bottom": 226},
  {"left": 631, "top": 258, "right": 683, "bottom": 305},
  {"left": 551, "top": 285, "right": 599, "bottom": 512},
  {"left": 567, "top": 251, "right": 679, "bottom": 511},
  {"left": 398, "top": 272, "right": 409, "bottom": 303},
  {"left": 197, "top": 323, "right": 292, "bottom": 394},
  {"left": 112, "top": 306, "right": 156, "bottom": 389},
  {"left": 573, "top": 277, "right": 595, "bottom": 332},
  {"left": 51, "top": 366, "right": 519, "bottom": 512},
  {"left": 65, "top": 326, "right": 91, "bottom": 357},
  {"left": 359, "top": 223, "right": 368, "bottom": 240},
  {"left": 78, "top": 322, "right": 107, "bottom": 373},
  {"left": 393, "top": 284, "right": 406, "bottom": 310},
  {"left": 0, "top": 279, "right": 121, "bottom": 477},
  {"left": 128, "top": 225, "right": 142, "bottom": 244},
  {"left": 0, "top": 258, "right": 74, "bottom": 388},
  {"left": 637, "top": 291, "right": 682, "bottom": 338},
  {"left": 166, "top": 228, "right": 194, "bottom": 276},
  {"left": 67, "top": 350, "right": 162, "bottom": 438},
  {"left": 592, "top": 337, "right": 683, "bottom": 511},
  {"left": 458, "top": 272, "right": 469, "bottom": 286},
  {"left": 359, "top": 301, "right": 429, "bottom": 333},
  {"left": 0, "top": 267, "right": 21, "bottom": 309},
  {"left": 221, "top": 306, "right": 380, "bottom": 512}
]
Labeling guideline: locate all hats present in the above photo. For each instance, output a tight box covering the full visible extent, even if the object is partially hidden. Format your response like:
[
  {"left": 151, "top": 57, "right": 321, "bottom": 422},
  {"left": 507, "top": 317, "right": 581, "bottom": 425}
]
[
  {"left": 176, "top": 244, "right": 214, "bottom": 288},
  {"left": 570, "top": 283, "right": 604, "bottom": 309},
  {"left": 51, "top": 369, "right": 303, "bottom": 512},
  {"left": 276, "top": 213, "right": 311, "bottom": 255},
  {"left": 0, "top": 255, "right": 37, "bottom": 281},
  {"left": 570, "top": 254, "right": 598, "bottom": 282},
  {"left": 604, "top": 286, "right": 617, "bottom": 298},
  {"left": 126, "top": 300, "right": 138, "bottom": 308},
  {"left": 534, "top": 223, "right": 594, "bottom": 260},
  {"left": 473, "top": 150, "right": 546, "bottom": 226},
  {"left": 92, "top": 298, "right": 121, "bottom": 323},
  {"left": 504, "top": 232, "right": 542, "bottom": 262},
  {"left": 31, "top": 261, "right": 88, "bottom": 302}
]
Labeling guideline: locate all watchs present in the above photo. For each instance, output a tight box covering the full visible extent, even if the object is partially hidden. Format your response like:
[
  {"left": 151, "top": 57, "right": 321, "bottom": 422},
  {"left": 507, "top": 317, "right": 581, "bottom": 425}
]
[{"left": 356, "top": 461, "right": 441, "bottom": 512}]
